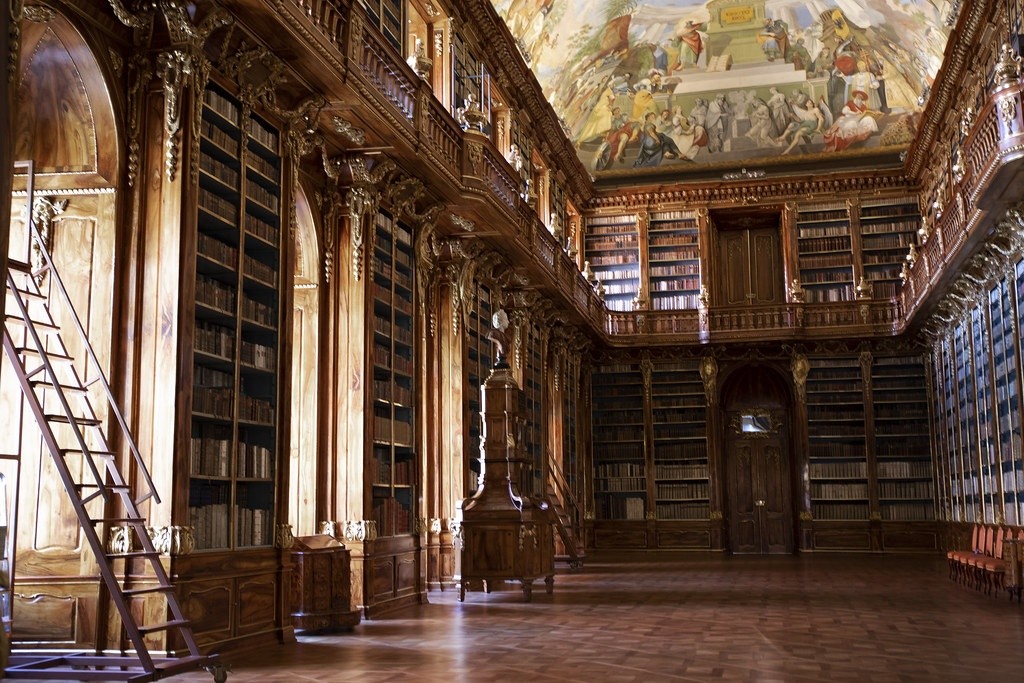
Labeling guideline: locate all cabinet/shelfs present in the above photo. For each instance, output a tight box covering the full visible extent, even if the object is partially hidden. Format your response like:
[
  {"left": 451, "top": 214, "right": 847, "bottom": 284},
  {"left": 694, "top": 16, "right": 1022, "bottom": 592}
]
[
  {"left": 366, "top": 182, "right": 422, "bottom": 615},
  {"left": 866, "top": 359, "right": 934, "bottom": 550},
  {"left": 557, "top": 347, "right": 579, "bottom": 560},
  {"left": 848, "top": 196, "right": 924, "bottom": 305},
  {"left": 583, "top": 350, "right": 650, "bottom": 561},
  {"left": 929, "top": 265, "right": 1021, "bottom": 525},
  {"left": 459, "top": 256, "right": 515, "bottom": 584},
  {"left": 640, "top": 207, "right": 704, "bottom": 318},
  {"left": 643, "top": 360, "right": 722, "bottom": 549},
  {"left": 577, "top": 203, "right": 647, "bottom": 317},
  {"left": 176, "top": 68, "right": 281, "bottom": 656},
  {"left": 784, "top": 196, "right": 861, "bottom": 307},
  {"left": 794, "top": 357, "right": 877, "bottom": 553},
  {"left": 525, "top": 313, "right": 549, "bottom": 565}
]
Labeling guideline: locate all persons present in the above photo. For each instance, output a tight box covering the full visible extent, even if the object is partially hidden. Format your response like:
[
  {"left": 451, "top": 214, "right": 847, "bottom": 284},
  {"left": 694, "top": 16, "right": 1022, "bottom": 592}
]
[{"left": 487, "top": 309, "right": 512, "bottom": 358}]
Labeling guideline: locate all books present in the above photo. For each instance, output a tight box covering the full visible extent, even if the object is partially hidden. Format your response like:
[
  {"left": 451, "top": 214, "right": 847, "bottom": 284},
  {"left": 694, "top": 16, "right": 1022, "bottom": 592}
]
[
  {"left": 803, "top": 355, "right": 936, "bottom": 519},
  {"left": 177, "top": 87, "right": 279, "bottom": 555},
  {"left": 793, "top": 196, "right": 922, "bottom": 307},
  {"left": 368, "top": 202, "right": 424, "bottom": 537},
  {"left": 559, "top": 356, "right": 715, "bottom": 522},
  {"left": 460, "top": 278, "right": 497, "bottom": 509},
  {"left": 522, "top": 321, "right": 544, "bottom": 498},
  {"left": 927, "top": 263, "right": 1024, "bottom": 528},
  {"left": 585, "top": 210, "right": 700, "bottom": 316}
]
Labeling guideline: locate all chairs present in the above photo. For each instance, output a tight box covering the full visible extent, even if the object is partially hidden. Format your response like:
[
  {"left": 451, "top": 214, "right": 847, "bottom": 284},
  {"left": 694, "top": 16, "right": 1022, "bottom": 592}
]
[{"left": 939, "top": 525, "right": 1023, "bottom": 598}]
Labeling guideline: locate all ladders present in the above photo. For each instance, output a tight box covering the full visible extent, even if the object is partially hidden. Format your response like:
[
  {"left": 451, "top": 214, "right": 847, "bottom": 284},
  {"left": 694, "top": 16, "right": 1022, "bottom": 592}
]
[
  {"left": 0, "top": 254, "right": 229, "bottom": 683},
  {"left": 547, "top": 446, "right": 586, "bottom": 570}
]
